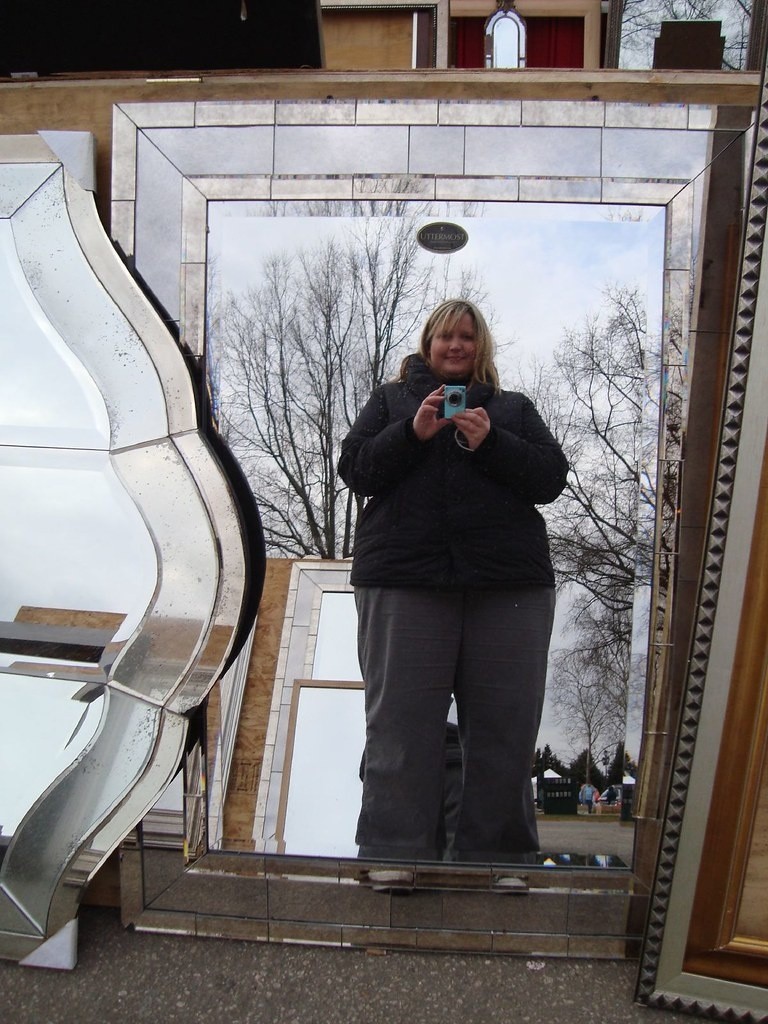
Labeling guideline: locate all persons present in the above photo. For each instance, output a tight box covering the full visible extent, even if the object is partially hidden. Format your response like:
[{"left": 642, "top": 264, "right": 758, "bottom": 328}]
[
  {"left": 336, "top": 297, "right": 571, "bottom": 896},
  {"left": 579, "top": 783, "right": 618, "bottom": 815}
]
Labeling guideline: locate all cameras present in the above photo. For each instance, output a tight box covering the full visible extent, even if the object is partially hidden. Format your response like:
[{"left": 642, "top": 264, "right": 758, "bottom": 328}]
[{"left": 444, "top": 386, "right": 466, "bottom": 419}]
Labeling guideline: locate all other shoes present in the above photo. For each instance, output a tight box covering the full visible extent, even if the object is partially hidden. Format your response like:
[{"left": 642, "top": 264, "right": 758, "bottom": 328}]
[
  {"left": 491, "top": 874, "right": 528, "bottom": 895},
  {"left": 368, "top": 866, "right": 415, "bottom": 895}
]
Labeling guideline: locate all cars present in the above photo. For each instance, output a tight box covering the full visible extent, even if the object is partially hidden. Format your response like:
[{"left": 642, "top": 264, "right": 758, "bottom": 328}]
[{"left": 597, "top": 785, "right": 622, "bottom": 806}]
